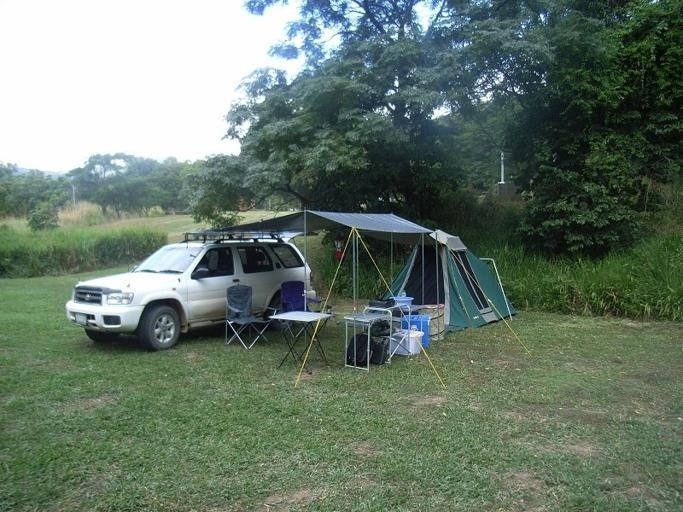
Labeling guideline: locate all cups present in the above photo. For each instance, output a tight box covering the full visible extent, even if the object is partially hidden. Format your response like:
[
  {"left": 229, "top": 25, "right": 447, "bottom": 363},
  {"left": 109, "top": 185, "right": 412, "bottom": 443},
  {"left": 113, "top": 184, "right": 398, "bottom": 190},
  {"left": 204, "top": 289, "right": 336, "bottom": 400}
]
[{"left": 411, "top": 325, "right": 417, "bottom": 336}]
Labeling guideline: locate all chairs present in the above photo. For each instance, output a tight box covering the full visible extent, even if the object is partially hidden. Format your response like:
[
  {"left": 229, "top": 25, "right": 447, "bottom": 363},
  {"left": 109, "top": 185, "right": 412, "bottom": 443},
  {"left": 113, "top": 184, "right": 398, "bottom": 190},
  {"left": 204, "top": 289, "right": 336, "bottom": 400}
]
[
  {"left": 226, "top": 284, "right": 278, "bottom": 350},
  {"left": 281, "top": 281, "right": 331, "bottom": 345}
]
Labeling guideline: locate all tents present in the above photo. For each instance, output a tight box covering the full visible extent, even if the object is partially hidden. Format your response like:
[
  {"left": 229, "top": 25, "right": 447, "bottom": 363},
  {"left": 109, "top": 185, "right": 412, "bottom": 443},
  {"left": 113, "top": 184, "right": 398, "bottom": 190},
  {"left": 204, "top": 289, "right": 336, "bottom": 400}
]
[{"left": 369, "top": 229, "right": 518, "bottom": 332}]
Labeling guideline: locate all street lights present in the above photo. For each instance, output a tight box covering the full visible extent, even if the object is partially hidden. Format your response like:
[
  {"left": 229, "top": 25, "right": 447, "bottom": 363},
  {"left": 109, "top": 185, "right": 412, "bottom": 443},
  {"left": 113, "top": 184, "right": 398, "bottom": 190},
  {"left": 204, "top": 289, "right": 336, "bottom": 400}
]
[
  {"left": 498, "top": 152, "right": 506, "bottom": 184},
  {"left": 56, "top": 172, "right": 75, "bottom": 222}
]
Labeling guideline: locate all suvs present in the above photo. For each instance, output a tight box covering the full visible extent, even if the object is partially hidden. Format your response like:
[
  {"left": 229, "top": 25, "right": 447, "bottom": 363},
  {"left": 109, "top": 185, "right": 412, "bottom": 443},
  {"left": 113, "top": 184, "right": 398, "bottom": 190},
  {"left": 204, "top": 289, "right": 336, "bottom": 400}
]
[{"left": 65, "top": 231, "right": 316, "bottom": 351}]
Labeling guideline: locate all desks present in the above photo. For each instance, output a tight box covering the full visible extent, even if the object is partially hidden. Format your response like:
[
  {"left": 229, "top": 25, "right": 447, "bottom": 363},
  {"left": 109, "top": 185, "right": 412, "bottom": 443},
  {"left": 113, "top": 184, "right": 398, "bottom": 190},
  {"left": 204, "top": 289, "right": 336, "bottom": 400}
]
[{"left": 269, "top": 311, "right": 332, "bottom": 375}]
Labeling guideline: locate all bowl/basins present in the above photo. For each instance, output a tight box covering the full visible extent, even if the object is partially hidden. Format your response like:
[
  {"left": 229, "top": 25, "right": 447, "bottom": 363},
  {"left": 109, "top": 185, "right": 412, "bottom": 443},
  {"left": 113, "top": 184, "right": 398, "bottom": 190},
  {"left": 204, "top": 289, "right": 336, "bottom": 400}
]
[{"left": 389, "top": 296, "right": 414, "bottom": 309}]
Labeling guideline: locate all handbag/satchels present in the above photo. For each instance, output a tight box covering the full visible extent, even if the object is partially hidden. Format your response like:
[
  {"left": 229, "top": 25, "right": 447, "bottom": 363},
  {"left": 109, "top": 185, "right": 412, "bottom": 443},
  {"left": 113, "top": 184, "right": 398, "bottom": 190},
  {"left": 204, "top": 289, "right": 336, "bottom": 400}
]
[
  {"left": 366, "top": 320, "right": 395, "bottom": 337},
  {"left": 369, "top": 298, "right": 395, "bottom": 307},
  {"left": 347, "top": 332, "right": 374, "bottom": 365}
]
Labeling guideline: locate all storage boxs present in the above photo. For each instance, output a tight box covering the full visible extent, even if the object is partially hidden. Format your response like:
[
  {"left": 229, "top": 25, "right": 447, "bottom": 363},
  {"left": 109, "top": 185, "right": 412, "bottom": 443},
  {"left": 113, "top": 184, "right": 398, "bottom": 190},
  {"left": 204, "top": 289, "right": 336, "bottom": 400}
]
[
  {"left": 401, "top": 315, "right": 430, "bottom": 347},
  {"left": 391, "top": 328, "right": 424, "bottom": 355}
]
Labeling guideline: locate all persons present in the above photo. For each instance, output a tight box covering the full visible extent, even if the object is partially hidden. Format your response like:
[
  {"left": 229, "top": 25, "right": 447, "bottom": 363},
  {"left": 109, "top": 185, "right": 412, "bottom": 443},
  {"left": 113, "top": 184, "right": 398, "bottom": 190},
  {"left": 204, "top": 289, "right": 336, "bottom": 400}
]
[{"left": 334, "top": 235, "right": 346, "bottom": 263}]
